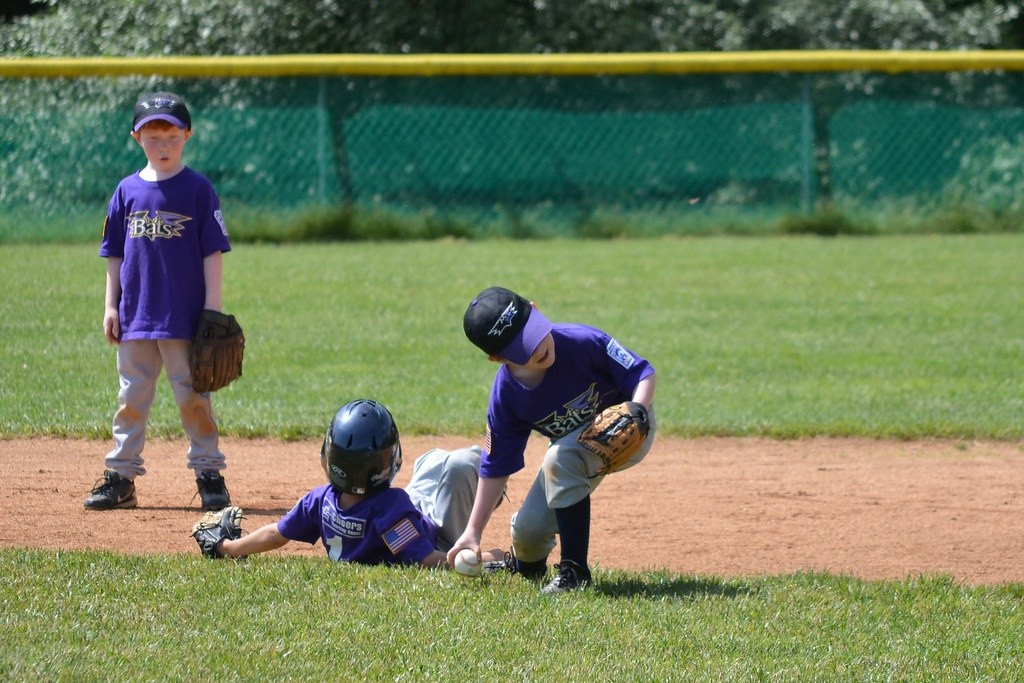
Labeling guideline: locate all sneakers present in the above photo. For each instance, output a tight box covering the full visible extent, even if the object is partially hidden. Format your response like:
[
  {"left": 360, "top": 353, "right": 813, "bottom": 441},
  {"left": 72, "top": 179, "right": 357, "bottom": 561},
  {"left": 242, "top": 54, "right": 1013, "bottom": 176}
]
[
  {"left": 483, "top": 546, "right": 547, "bottom": 581},
  {"left": 84, "top": 470, "right": 137, "bottom": 510},
  {"left": 539, "top": 559, "right": 591, "bottom": 595},
  {"left": 189, "top": 472, "right": 232, "bottom": 510}
]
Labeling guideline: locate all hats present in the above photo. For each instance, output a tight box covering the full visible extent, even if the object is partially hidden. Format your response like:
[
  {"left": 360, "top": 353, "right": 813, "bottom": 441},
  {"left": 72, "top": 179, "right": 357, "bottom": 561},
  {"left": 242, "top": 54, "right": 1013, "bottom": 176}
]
[
  {"left": 132, "top": 92, "right": 191, "bottom": 133},
  {"left": 464, "top": 287, "right": 553, "bottom": 365}
]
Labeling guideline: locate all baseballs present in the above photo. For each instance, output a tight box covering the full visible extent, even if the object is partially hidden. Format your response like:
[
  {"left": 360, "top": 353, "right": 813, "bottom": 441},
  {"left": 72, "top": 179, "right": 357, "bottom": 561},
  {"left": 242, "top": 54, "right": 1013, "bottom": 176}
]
[{"left": 454, "top": 548, "right": 483, "bottom": 577}]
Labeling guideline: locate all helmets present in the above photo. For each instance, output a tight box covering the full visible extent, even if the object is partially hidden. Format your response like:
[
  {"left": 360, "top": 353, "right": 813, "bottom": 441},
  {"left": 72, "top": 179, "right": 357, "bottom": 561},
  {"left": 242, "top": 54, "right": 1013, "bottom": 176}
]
[{"left": 320, "top": 399, "right": 403, "bottom": 495}]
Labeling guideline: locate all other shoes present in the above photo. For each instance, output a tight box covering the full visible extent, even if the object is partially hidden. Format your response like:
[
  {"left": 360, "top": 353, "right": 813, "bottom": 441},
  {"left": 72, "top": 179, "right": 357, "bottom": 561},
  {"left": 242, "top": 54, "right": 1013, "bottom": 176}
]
[{"left": 492, "top": 484, "right": 511, "bottom": 511}]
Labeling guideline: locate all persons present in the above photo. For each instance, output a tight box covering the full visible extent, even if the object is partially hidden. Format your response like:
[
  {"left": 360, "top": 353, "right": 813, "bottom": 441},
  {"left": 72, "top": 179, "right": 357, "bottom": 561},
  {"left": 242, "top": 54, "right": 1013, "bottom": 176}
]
[
  {"left": 446, "top": 286, "right": 656, "bottom": 594},
  {"left": 83, "top": 92, "right": 232, "bottom": 509},
  {"left": 190, "top": 399, "right": 505, "bottom": 568}
]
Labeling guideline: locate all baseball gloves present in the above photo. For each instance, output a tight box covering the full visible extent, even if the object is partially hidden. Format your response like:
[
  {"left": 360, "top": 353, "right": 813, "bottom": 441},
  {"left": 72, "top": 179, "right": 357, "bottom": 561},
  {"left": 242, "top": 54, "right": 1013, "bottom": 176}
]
[
  {"left": 187, "top": 309, "right": 247, "bottom": 397},
  {"left": 576, "top": 399, "right": 652, "bottom": 476},
  {"left": 190, "top": 505, "right": 248, "bottom": 559}
]
